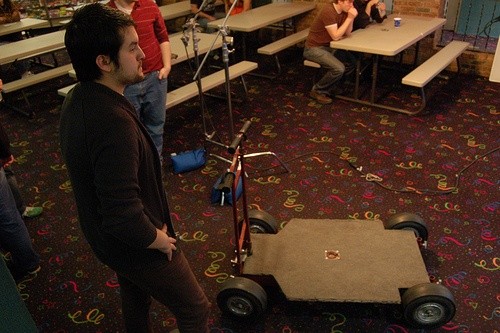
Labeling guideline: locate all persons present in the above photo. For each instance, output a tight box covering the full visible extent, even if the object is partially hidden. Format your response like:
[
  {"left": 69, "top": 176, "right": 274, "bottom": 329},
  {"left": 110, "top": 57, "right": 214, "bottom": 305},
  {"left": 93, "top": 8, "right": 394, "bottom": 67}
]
[
  {"left": 0, "top": 108, "right": 43, "bottom": 287},
  {"left": 59, "top": 4, "right": 215, "bottom": 333},
  {"left": 191, "top": 0, "right": 388, "bottom": 106},
  {"left": 102, "top": 0, "right": 172, "bottom": 171}
]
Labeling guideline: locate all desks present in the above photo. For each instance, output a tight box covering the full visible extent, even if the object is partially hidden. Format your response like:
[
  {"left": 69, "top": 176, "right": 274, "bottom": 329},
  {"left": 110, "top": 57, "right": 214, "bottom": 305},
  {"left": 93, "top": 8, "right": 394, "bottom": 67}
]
[
  {"left": 158, "top": 0, "right": 224, "bottom": 21},
  {"left": 329, "top": 13, "right": 447, "bottom": 107},
  {"left": 206, "top": 1, "right": 316, "bottom": 77},
  {"left": 0, "top": 29, "right": 66, "bottom": 109},
  {"left": 0, "top": 17, "right": 48, "bottom": 38},
  {"left": 68, "top": 32, "right": 234, "bottom": 99}
]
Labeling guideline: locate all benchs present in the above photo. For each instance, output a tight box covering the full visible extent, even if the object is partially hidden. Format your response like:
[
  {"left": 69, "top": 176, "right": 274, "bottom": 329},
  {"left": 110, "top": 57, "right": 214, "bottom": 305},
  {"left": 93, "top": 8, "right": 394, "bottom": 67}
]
[
  {"left": 257, "top": 27, "right": 310, "bottom": 79},
  {"left": 303, "top": 60, "right": 321, "bottom": 86},
  {"left": 166, "top": 60, "right": 258, "bottom": 110},
  {"left": 2, "top": 63, "right": 73, "bottom": 117},
  {"left": 402, "top": 40, "right": 470, "bottom": 114}
]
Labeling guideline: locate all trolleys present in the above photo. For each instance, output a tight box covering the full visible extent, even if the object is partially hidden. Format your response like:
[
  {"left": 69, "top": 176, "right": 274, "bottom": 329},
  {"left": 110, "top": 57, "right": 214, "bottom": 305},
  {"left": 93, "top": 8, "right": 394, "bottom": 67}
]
[{"left": 210, "top": 120, "right": 455, "bottom": 330}]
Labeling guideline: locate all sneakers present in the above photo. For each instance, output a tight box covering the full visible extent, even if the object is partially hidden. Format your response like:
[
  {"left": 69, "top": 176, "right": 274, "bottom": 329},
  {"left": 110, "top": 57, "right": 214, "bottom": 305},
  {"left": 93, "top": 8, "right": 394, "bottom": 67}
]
[
  {"left": 308, "top": 86, "right": 333, "bottom": 104},
  {"left": 22, "top": 205, "right": 45, "bottom": 217},
  {"left": 28, "top": 265, "right": 42, "bottom": 274}
]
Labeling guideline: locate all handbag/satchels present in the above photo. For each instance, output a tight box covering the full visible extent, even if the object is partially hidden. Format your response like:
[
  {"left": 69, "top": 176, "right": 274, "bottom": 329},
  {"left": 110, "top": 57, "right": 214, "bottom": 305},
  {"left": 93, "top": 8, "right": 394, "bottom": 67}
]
[
  {"left": 211, "top": 171, "right": 244, "bottom": 205},
  {"left": 170, "top": 147, "right": 207, "bottom": 175}
]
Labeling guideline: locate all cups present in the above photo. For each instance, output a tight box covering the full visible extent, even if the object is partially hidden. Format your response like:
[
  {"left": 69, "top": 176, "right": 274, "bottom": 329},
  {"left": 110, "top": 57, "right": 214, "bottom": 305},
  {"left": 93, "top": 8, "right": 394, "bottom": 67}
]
[{"left": 393, "top": 18, "right": 402, "bottom": 28}]
